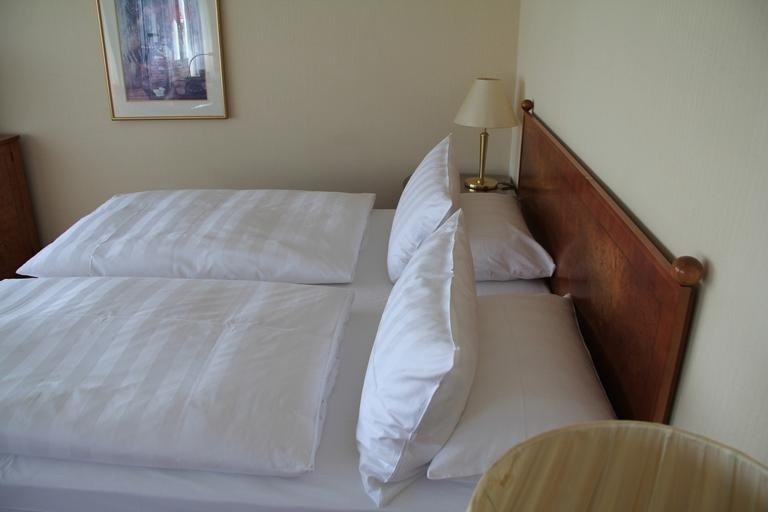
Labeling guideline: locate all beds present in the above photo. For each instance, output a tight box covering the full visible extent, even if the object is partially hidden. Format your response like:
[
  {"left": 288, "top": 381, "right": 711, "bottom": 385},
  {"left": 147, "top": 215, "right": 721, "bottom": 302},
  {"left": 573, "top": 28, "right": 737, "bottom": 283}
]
[{"left": 0, "top": 98, "right": 704, "bottom": 512}]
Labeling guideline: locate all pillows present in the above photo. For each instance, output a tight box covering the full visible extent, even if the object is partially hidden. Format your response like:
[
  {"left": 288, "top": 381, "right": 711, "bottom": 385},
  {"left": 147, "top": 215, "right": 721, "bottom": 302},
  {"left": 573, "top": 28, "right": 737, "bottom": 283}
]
[
  {"left": 355, "top": 209, "right": 478, "bottom": 507},
  {"left": 459, "top": 193, "right": 557, "bottom": 282},
  {"left": 386, "top": 133, "right": 462, "bottom": 282},
  {"left": 426, "top": 291, "right": 618, "bottom": 481}
]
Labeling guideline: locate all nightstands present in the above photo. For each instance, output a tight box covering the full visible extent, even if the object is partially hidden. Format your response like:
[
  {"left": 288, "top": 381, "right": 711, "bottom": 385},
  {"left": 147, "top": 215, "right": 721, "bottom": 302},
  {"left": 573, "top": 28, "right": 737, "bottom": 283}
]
[{"left": 404, "top": 173, "right": 514, "bottom": 193}]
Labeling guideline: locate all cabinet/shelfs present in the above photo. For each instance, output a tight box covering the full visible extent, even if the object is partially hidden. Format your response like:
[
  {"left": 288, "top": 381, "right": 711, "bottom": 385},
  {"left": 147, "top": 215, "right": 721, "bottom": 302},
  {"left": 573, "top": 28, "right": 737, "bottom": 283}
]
[{"left": 0, "top": 134, "right": 40, "bottom": 279}]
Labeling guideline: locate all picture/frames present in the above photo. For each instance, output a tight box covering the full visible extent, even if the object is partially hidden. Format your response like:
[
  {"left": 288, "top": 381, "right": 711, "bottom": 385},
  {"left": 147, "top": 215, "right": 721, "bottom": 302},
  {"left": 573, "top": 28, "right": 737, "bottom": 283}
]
[{"left": 95, "top": 0, "right": 229, "bottom": 121}]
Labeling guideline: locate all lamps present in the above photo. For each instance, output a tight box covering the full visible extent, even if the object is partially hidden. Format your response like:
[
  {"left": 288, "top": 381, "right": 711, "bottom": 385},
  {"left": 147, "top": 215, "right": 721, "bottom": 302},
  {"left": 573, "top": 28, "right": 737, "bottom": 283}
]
[
  {"left": 467, "top": 420, "right": 768, "bottom": 512},
  {"left": 453, "top": 78, "right": 520, "bottom": 191}
]
